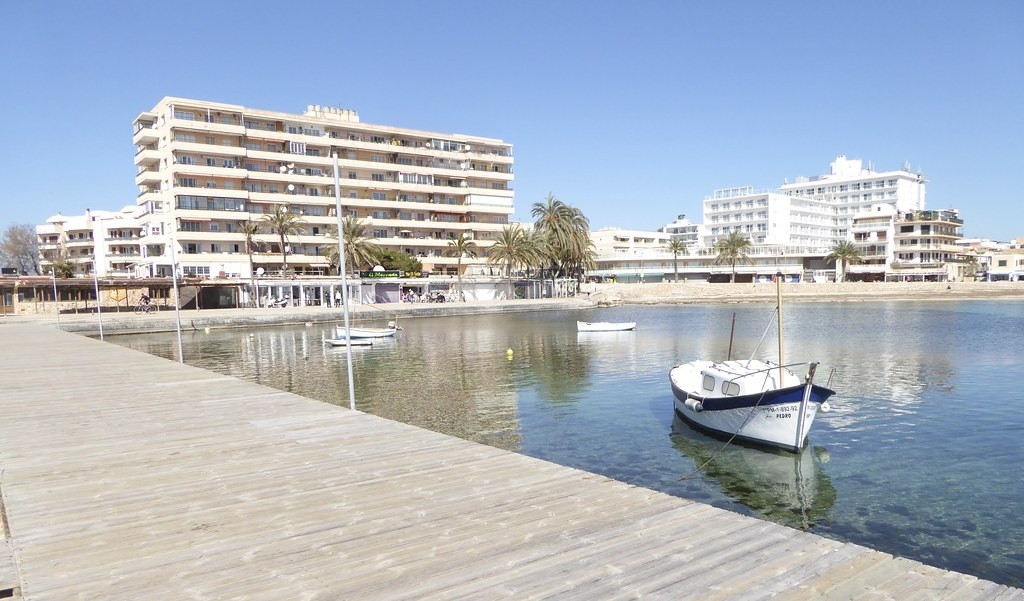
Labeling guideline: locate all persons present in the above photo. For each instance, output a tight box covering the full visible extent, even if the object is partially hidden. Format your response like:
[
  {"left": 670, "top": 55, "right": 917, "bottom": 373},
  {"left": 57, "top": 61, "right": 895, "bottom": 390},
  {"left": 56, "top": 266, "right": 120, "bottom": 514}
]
[
  {"left": 138, "top": 293, "right": 150, "bottom": 311},
  {"left": 407, "top": 288, "right": 466, "bottom": 303},
  {"left": 306, "top": 288, "right": 342, "bottom": 308}
]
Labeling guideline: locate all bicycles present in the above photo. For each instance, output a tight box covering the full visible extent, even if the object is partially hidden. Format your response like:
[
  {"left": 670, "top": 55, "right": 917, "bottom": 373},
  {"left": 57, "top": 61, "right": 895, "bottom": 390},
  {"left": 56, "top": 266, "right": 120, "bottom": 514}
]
[{"left": 133, "top": 301, "right": 157, "bottom": 315}]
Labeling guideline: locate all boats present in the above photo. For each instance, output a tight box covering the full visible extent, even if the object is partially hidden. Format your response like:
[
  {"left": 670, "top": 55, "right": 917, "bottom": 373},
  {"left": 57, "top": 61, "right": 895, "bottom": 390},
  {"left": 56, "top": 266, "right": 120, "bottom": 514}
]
[
  {"left": 598, "top": 298, "right": 624, "bottom": 308},
  {"left": 334, "top": 322, "right": 400, "bottom": 339},
  {"left": 669, "top": 277, "right": 838, "bottom": 455},
  {"left": 575, "top": 320, "right": 636, "bottom": 332},
  {"left": 324, "top": 338, "right": 374, "bottom": 346}
]
